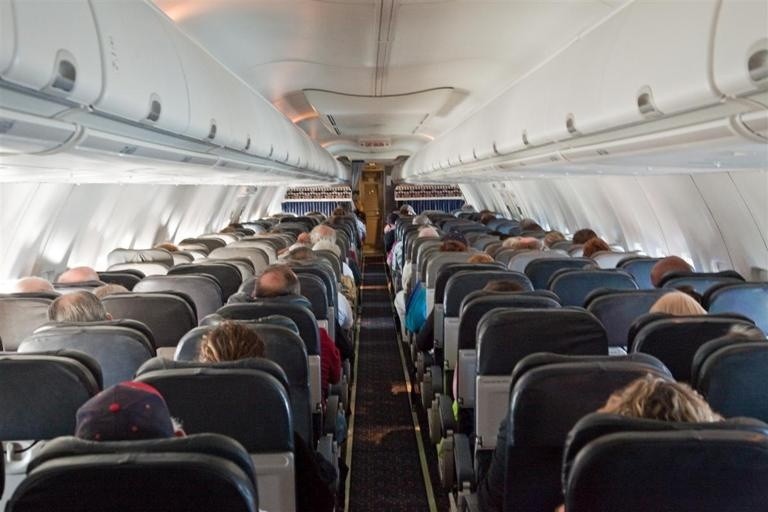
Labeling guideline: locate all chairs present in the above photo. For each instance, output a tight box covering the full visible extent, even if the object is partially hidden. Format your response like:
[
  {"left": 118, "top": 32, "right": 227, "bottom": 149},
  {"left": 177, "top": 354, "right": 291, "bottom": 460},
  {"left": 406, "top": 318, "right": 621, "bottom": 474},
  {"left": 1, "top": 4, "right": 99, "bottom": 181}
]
[{"left": 0, "top": 210, "right": 768, "bottom": 512}]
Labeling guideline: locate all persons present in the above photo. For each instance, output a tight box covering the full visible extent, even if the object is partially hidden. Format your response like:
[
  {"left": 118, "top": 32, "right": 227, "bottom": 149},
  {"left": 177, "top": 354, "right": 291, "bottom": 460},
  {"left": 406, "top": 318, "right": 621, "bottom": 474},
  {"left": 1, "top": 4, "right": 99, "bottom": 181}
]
[
  {"left": 384, "top": 206, "right": 415, "bottom": 253},
  {"left": 418, "top": 254, "right": 494, "bottom": 354},
  {"left": 504, "top": 236, "right": 541, "bottom": 249},
  {"left": 311, "top": 224, "right": 355, "bottom": 280},
  {"left": 13, "top": 278, "right": 51, "bottom": 291},
  {"left": 649, "top": 293, "right": 707, "bottom": 315},
  {"left": 59, "top": 266, "right": 98, "bottom": 282},
  {"left": 48, "top": 291, "right": 112, "bottom": 321},
  {"left": 583, "top": 238, "right": 609, "bottom": 257},
  {"left": 324, "top": 207, "right": 366, "bottom": 259},
  {"left": 288, "top": 248, "right": 354, "bottom": 328},
  {"left": 314, "top": 241, "right": 356, "bottom": 301},
  {"left": 94, "top": 284, "right": 128, "bottom": 298},
  {"left": 520, "top": 219, "right": 542, "bottom": 231},
  {"left": 156, "top": 238, "right": 209, "bottom": 252},
  {"left": 480, "top": 211, "right": 492, "bottom": 224},
  {"left": 478, "top": 417, "right": 509, "bottom": 512},
  {"left": 462, "top": 206, "right": 473, "bottom": 211},
  {"left": 254, "top": 264, "right": 341, "bottom": 408},
  {"left": 387, "top": 216, "right": 467, "bottom": 320},
  {"left": 75, "top": 382, "right": 184, "bottom": 440},
  {"left": 600, "top": 378, "right": 720, "bottom": 422},
  {"left": 650, "top": 256, "right": 692, "bottom": 287},
  {"left": 544, "top": 231, "right": 565, "bottom": 251},
  {"left": 200, "top": 320, "right": 337, "bottom": 512},
  {"left": 223, "top": 223, "right": 280, "bottom": 233},
  {"left": 453, "top": 281, "right": 525, "bottom": 421},
  {"left": 574, "top": 229, "right": 597, "bottom": 243},
  {"left": 405, "top": 241, "right": 466, "bottom": 332}
]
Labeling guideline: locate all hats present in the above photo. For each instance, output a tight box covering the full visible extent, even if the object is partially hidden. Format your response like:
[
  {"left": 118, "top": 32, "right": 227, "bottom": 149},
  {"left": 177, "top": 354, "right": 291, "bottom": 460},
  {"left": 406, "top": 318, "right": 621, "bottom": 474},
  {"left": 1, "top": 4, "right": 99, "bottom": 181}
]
[{"left": 76, "top": 382, "right": 175, "bottom": 441}]
